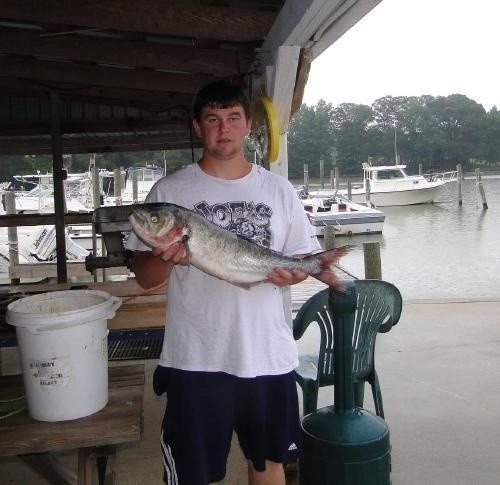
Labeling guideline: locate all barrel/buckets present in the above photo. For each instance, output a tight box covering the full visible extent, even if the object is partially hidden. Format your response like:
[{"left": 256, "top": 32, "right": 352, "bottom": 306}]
[{"left": 7, "top": 289, "right": 123, "bottom": 423}]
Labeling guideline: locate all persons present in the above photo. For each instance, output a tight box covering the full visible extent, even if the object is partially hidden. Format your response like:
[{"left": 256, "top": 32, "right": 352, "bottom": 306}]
[{"left": 124, "top": 80, "right": 324, "bottom": 485}]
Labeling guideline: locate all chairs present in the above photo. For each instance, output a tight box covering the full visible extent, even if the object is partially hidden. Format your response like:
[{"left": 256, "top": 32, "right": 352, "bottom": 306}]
[{"left": 292, "top": 280, "right": 403, "bottom": 419}]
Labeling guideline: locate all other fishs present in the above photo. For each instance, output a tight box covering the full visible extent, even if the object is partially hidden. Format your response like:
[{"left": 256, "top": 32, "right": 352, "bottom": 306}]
[{"left": 127, "top": 202, "right": 357, "bottom": 291}]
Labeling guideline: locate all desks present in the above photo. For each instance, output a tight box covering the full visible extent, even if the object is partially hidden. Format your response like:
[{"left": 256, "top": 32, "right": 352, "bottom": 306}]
[{"left": 0, "top": 363, "right": 145, "bottom": 485}]
